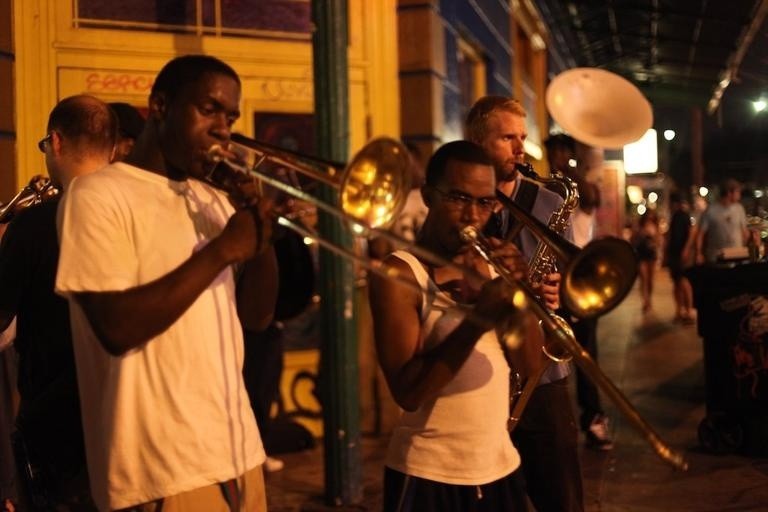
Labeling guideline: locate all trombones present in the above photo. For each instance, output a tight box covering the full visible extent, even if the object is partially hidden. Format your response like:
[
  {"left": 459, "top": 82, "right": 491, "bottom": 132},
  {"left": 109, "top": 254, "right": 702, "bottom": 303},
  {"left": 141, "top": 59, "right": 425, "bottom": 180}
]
[
  {"left": 198, "top": 131, "right": 525, "bottom": 336},
  {"left": 456, "top": 187, "right": 690, "bottom": 474}
]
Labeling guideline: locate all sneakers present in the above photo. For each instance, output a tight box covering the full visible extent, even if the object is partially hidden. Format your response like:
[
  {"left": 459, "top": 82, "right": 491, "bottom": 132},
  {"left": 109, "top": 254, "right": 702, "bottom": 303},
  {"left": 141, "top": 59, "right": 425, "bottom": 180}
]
[
  {"left": 262, "top": 455, "right": 284, "bottom": 471},
  {"left": 587, "top": 415, "right": 613, "bottom": 450}
]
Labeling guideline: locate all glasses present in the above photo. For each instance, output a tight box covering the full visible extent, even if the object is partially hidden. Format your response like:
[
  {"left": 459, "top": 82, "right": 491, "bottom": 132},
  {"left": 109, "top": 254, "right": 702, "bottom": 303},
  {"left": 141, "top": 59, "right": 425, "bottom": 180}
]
[
  {"left": 38, "top": 131, "right": 61, "bottom": 153},
  {"left": 434, "top": 186, "right": 497, "bottom": 213}
]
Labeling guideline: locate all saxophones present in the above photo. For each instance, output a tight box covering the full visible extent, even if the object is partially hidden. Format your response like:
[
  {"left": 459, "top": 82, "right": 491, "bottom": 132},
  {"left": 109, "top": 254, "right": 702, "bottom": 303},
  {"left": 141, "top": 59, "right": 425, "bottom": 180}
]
[{"left": 506, "top": 159, "right": 582, "bottom": 433}]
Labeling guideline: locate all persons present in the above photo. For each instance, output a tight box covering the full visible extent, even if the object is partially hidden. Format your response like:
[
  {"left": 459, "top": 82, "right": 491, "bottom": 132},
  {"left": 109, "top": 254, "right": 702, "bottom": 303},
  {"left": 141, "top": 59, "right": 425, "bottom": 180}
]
[
  {"left": 59, "top": 54, "right": 279, "bottom": 512},
  {"left": 0, "top": 93, "right": 119, "bottom": 512},
  {"left": 541, "top": 131, "right": 614, "bottom": 451},
  {"left": 681, "top": 183, "right": 707, "bottom": 309},
  {"left": 695, "top": 179, "right": 752, "bottom": 358},
  {"left": 367, "top": 140, "right": 526, "bottom": 512},
  {"left": 464, "top": 93, "right": 584, "bottom": 512},
  {"left": 663, "top": 190, "right": 696, "bottom": 323},
  {"left": 629, "top": 207, "right": 661, "bottom": 314},
  {"left": 109, "top": 102, "right": 146, "bottom": 163}
]
